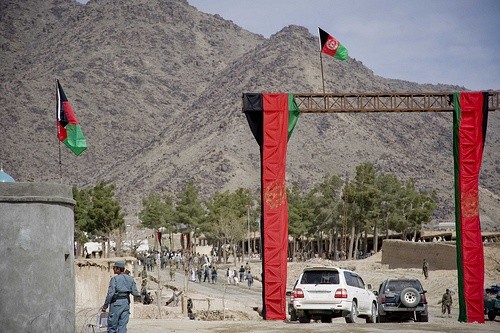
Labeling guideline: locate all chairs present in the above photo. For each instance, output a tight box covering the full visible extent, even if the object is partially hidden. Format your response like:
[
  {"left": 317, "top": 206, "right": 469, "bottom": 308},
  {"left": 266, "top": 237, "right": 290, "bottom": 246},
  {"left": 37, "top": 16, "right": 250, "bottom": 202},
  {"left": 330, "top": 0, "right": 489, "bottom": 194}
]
[
  {"left": 389, "top": 286, "right": 395, "bottom": 291},
  {"left": 329, "top": 276, "right": 336, "bottom": 282}
]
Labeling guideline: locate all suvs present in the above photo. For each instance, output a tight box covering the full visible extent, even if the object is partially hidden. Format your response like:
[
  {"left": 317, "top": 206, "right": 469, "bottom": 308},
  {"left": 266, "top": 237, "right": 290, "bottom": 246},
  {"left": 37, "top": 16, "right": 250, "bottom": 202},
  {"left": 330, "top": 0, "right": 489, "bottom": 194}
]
[
  {"left": 375, "top": 277, "right": 429, "bottom": 323},
  {"left": 286, "top": 265, "right": 379, "bottom": 323}
]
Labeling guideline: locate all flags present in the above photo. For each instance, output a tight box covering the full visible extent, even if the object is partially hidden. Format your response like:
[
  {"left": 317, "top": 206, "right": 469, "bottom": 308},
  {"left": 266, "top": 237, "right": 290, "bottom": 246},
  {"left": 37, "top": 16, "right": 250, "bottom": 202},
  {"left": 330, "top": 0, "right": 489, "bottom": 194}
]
[
  {"left": 318, "top": 27, "right": 348, "bottom": 62},
  {"left": 56, "top": 79, "right": 88, "bottom": 156}
]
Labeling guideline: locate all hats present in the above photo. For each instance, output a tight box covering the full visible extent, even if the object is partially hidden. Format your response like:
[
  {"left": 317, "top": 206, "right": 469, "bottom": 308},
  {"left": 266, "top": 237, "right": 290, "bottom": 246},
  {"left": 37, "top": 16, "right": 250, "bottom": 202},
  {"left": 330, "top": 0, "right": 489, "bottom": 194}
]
[{"left": 113, "top": 261, "right": 124, "bottom": 268}]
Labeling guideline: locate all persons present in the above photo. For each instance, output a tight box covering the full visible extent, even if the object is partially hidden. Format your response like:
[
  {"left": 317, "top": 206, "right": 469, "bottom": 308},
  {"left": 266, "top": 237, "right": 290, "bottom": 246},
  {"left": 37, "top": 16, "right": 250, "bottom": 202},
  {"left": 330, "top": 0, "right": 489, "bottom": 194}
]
[
  {"left": 138, "top": 249, "right": 253, "bottom": 287},
  {"left": 422, "top": 259, "right": 428, "bottom": 280},
  {"left": 484, "top": 236, "right": 493, "bottom": 242},
  {"left": 101, "top": 261, "right": 142, "bottom": 333},
  {"left": 411, "top": 236, "right": 447, "bottom": 243},
  {"left": 441, "top": 289, "right": 453, "bottom": 318},
  {"left": 294, "top": 249, "right": 360, "bottom": 263}
]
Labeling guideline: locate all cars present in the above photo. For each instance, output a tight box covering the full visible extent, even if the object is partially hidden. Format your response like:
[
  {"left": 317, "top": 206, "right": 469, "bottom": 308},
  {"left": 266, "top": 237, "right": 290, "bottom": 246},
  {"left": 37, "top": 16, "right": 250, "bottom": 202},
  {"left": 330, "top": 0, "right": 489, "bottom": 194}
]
[{"left": 484, "top": 284, "right": 500, "bottom": 322}]
